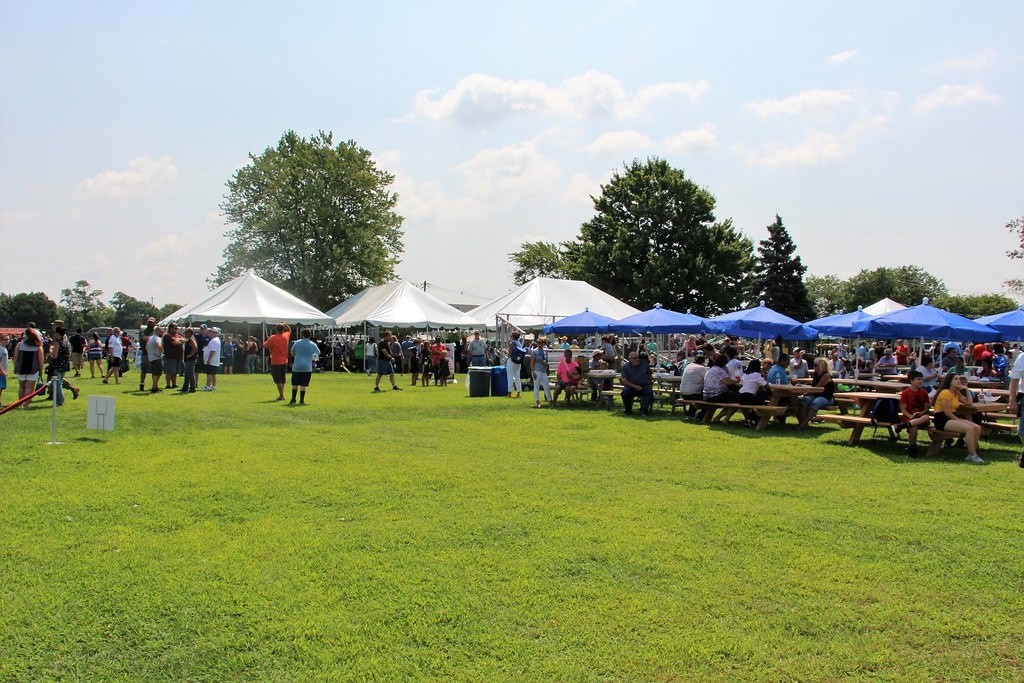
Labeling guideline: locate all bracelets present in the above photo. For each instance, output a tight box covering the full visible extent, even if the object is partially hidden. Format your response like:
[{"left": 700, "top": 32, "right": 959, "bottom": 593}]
[{"left": 389, "top": 357, "right": 393, "bottom": 360}]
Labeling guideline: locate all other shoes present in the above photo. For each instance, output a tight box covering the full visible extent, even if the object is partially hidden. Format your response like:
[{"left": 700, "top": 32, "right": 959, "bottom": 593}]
[
  {"left": 172, "top": 386, "right": 177, "bottom": 387},
  {"left": 103, "top": 380, "right": 108, "bottom": 384},
  {"left": 1019, "top": 452, "right": 1024, "bottom": 468},
  {"left": 744, "top": 419, "right": 757, "bottom": 427},
  {"left": 393, "top": 386, "right": 402, "bottom": 390},
  {"left": 73, "top": 388, "right": 79, "bottom": 399},
  {"left": 812, "top": 418, "right": 825, "bottom": 424},
  {"left": 166, "top": 386, "right": 170, "bottom": 388},
  {"left": 967, "top": 454, "right": 984, "bottom": 462},
  {"left": 374, "top": 387, "right": 380, "bottom": 392},
  {"left": 75, "top": 371, "right": 81, "bottom": 377},
  {"left": 45, "top": 397, "right": 53, "bottom": 401},
  {"left": 200, "top": 386, "right": 216, "bottom": 391},
  {"left": 151, "top": 389, "right": 159, "bottom": 392},
  {"left": 891, "top": 424, "right": 901, "bottom": 437},
  {"left": 140, "top": 384, "right": 144, "bottom": 390}
]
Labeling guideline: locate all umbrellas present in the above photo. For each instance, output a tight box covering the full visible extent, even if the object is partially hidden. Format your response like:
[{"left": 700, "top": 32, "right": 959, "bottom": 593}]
[{"left": 543, "top": 297, "right": 1024, "bottom": 417}]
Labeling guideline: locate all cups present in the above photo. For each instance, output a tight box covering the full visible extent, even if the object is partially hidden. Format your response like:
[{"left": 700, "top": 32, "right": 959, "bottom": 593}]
[
  {"left": 670, "top": 371, "right": 674, "bottom": 376},
  {"left": 735, "top": 371, "right": 740, "bottom": 380},
  {"left": 791, "top": 375, "right": 798, "bottom": 386}
]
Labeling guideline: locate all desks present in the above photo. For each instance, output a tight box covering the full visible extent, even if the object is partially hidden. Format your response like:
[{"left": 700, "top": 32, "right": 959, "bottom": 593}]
[
  {"left": 856, "top": 373, "right": 879, "bottom": 377},
  {"left": 833, "top": 392, "right": 1009, "bottom": 413},
  {"left": 881, "top": 375, "right": 907, "bottom": 381},
  {"left": 807, "top": 369, "right": 838, "bottom": 376},
  {"left": 969, "top": 387, "right": 1018, "bottom": 398},
  {"left": 733, "top": 384, "right": 824, "bottom": 394},
  {"left": 792, "top": 377, "right": 910, "bottom": 390},
  {"left": 649, "top": 367, "right": 667, "bottom": 372},
  {"left": 616, "top": 373, "right": 682, "bottom": 383},
  {"left": 968, "top": 381, "right": 1000, "bottom": 387},
  {"left": 581, "top": 373, "right": 621, "bottom": 379}
]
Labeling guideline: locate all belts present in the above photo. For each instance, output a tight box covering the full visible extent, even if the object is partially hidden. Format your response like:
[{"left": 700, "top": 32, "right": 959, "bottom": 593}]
[{"left": 473, "top": 354, "right": 484, "bottom": 357}]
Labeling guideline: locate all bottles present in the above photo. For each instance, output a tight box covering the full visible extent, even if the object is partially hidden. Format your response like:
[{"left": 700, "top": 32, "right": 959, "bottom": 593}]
[
  {"left": 979, "top": 388, "right": 985, "bottom": 405},
  {"left": 776, "top": 373, "right": 780, "bottom": 385}
]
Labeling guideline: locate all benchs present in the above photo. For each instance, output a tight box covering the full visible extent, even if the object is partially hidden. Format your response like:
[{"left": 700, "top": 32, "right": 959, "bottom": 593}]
[{"left": 545, "top": 361, "right": 1024, "bottom": 450}]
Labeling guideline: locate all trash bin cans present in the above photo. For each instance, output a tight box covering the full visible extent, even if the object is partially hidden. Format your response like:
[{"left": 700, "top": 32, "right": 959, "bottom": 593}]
[
  {"left": 490, "top": 366, "right": 508, "bottom": 397},
  {"left": 468, "top": 367, "right": 490, "bottom": 397}
]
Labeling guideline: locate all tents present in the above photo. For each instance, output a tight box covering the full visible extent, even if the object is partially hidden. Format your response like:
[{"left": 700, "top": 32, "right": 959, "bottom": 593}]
[
  {"left": 462, "top": 276, "right": 645, "bottom": 346},
  {"left": 300, "top": 279, "right": 486, "bottom": 372},
  {"left": 156, "top": 270, "right": 335, "bottom": 372}
]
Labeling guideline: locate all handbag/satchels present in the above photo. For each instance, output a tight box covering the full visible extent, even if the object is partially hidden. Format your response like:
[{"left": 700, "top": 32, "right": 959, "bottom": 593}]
[
  {"left": 546, "top": 365, "right": 549, "bottom": 376},
  {"left": 120, "top": 360, "right": 130, "bottom": 373},
  {"left": 36, "top": 377, "right": 45, "bottom": 395},
  {"left": 511, "top": 347, "right": 527, "bottom": 364}
]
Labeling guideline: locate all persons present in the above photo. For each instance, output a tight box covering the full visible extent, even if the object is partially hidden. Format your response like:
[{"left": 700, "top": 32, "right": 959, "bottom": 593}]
[
  {"left": 0, "top": 317, "right": 221, "bottom": 408},
  {"left": 530, "top": 331, "right": 1024, "bottom": 469},
  {"left": 218, "top": 329, "right": 549, "bottom": 400},
  {"left": 263, "top": 322, "right": 291, "bottom": 400},
  {"left": 290, "top": 328, "right": 321, "bottom": 404}
]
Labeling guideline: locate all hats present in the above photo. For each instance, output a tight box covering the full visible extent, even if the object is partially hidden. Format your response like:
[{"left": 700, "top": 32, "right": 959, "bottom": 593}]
[
  {"left": 208, "top": 327, "right": 221, "bottom": 332},
  {"left": 592, "top": 349, "right": 604, "bottom": 357},
  {"left": 51, "top": 320, "right": 64, "bottom": 325},
  {"left": 792, "top": 347, "right": 801, "bottom": 353},
  {"left": 200, "top": 324, "right": 207, "bottom": 328},
  {"left": 147, "top": 317, "right": 155, "bottom": 322}
]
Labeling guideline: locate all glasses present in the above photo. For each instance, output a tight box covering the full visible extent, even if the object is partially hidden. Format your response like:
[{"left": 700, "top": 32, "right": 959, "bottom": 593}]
[
  {"left": 537, "top": 342, "right": 544, "bottom": 344},
  {"left": 174, "top": 328, "right": 178, "bottom": 329},
  {"left": 634, "top": 356, "right": 639, "bottom": 360}
]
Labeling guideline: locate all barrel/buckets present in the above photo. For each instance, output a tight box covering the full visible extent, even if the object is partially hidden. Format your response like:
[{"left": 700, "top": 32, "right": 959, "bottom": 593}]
[
  {"left": 468, "top": 367, "right": 492, "bottom": 397},
  {"left": 491, "top": 368, "right": 508, "bottom": 396}
]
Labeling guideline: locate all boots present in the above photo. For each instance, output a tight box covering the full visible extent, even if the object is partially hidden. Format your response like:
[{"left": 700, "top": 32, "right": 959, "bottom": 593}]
[{"left": 290, "top": 389, "right": 306, "bottom": 404}]
